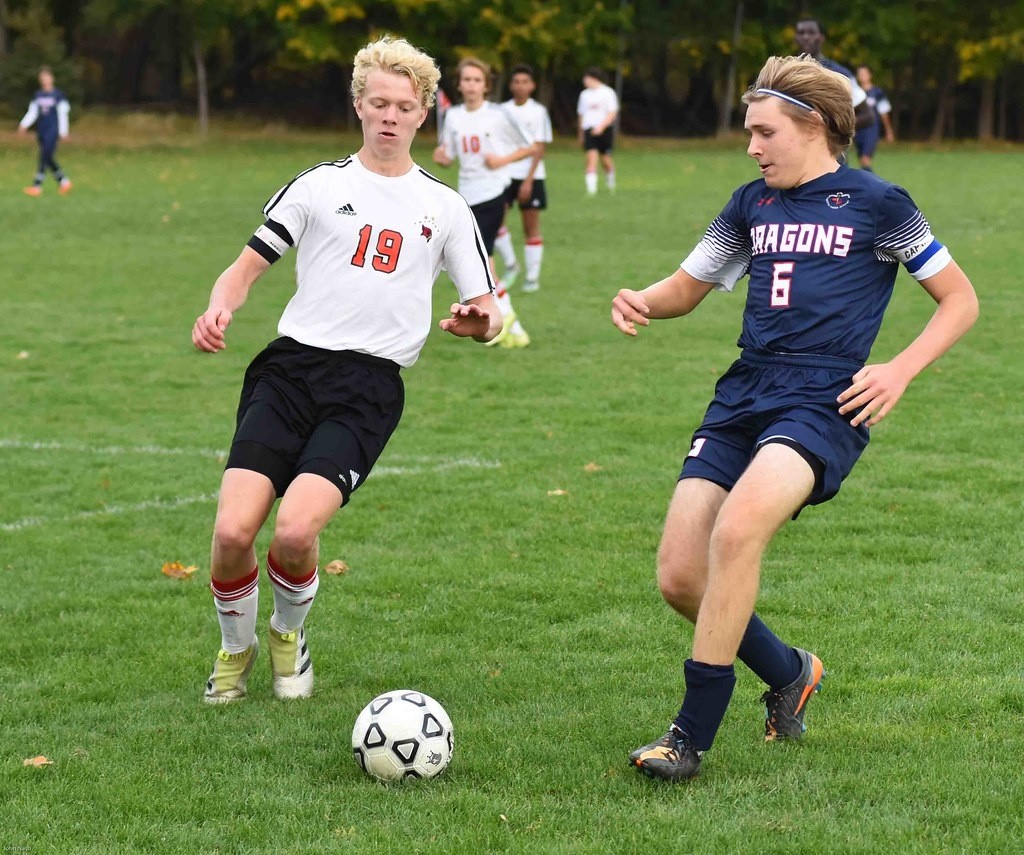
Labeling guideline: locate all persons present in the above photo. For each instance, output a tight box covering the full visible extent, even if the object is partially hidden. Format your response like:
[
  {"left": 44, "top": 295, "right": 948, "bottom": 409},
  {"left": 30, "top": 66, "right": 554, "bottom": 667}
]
[
  {"left": 193, "top": 39, "right": 504, "bottom": 705},
  {"left": 18, "top": 69, "right": 72, "bottom": 195},
  {"left": 577, "top": 71, "right": 620, "bottom": 194},
  {"left": 795, "top": 17, "right": 874, "bottom": 131},
  {"left": 855, "top": 65, "right": 893, "bottom": 172},
  {"left": 495, "top": 67, "right": 553, "bottom": 291},
  {"left": 432, "top": 59, "right": 536, "bottom": 347},
  {"left": 612, "top": 58, "right": 980, "bottom": 782}
]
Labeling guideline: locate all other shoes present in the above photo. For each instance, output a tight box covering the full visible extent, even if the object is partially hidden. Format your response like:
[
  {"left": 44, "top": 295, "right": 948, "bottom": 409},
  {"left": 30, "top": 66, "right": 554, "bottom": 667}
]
[
  {"left": 22, "top": 187, "right": 41, "bottom": 197},
  {"left": 58, "top": 181, "right": 73, "bottom": 193},
  {"left": 521, "top": 278, "right": 543, "bottom": 292},
  {"left": 485, "top": 310, "right": 530, "bottom": 350},
  {"left": 497, "top": 261, "right": 520, "bottom": 290}
]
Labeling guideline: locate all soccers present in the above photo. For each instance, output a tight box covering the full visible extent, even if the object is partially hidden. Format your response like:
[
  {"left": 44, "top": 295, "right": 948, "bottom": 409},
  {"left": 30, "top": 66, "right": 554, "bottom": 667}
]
[{"left": 351, "top": 688, "right": 457, "bottom": 789}]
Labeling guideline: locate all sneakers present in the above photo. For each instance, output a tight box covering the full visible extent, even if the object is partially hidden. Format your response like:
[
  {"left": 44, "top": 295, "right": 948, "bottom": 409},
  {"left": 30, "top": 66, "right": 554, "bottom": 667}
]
[
  {"left": 628, "top": 722, "right": 704, "bottom": 783},
  {"left": 268, "top": 615, "right": 313, "bottom": 702},
  {"left": 759, "top": 647, "right": 826, "bottom": 744},
  {"left": 203, "top": 633, "right": 259, "bottom": 705}
]
